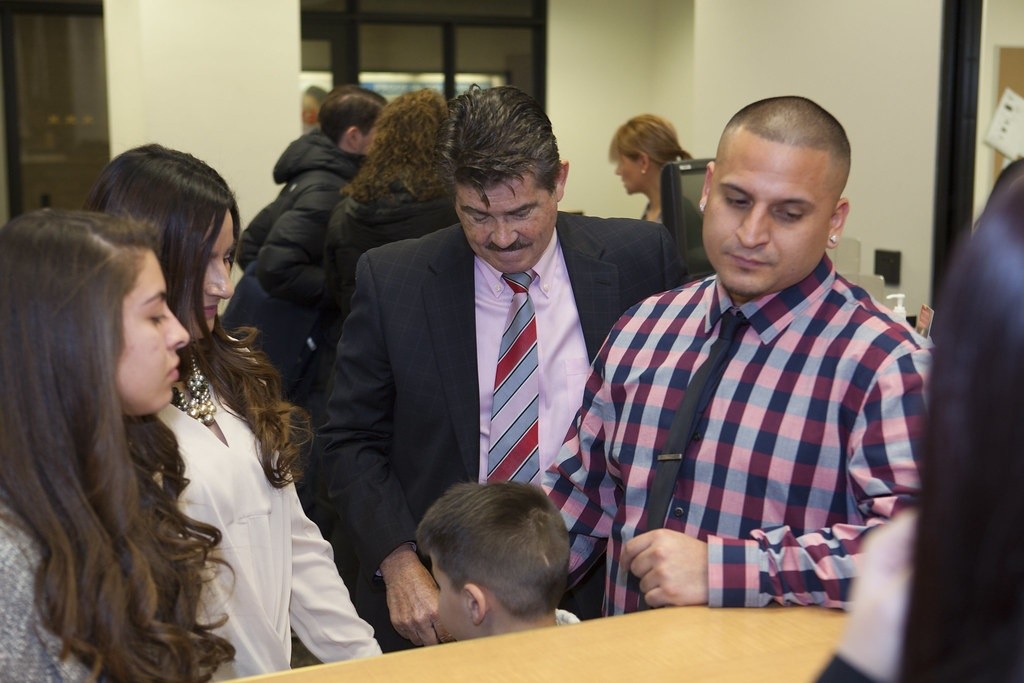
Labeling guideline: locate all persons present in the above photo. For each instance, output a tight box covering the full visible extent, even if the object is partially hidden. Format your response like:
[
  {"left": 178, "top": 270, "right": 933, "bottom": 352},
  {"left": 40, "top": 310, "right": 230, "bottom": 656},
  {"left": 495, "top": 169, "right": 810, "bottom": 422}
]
[
  {"left": 810, "top": 158, "right": 1024, "bottom": 682},
  {"left": 85, "top": 141, "right": 383, "bottom": 680},
  {"left": 221, "top": 76, "right": 717, "bottom": 523},
  {"left": 0, "top": 207, "right": 237, "bottom": 683},
  {"left": 547, "top": 97, "right": 935, "bottom": 621},
  {"left": 414, "top": 478, "right": 583, "bottom": 639},
  {"left": 327, "top": 83, "right": 681, "bottom": 652}
]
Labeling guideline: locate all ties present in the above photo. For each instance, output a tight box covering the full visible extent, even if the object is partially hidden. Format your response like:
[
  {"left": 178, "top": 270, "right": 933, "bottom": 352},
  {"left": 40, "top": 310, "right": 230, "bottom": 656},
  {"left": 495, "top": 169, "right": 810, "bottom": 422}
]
[
  {"left": 487, "top": 270, "right": 545, "bottom": 486},
  {"left": 644, "top": 309, "right": 750, "bottom": 533}
]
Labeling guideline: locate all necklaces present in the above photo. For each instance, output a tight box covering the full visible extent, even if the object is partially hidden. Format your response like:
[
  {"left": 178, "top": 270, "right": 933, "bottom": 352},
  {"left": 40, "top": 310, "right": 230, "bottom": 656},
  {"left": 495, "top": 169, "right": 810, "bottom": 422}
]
[{"left": 157, "top": 344, "right": 218, "bottom": 429}]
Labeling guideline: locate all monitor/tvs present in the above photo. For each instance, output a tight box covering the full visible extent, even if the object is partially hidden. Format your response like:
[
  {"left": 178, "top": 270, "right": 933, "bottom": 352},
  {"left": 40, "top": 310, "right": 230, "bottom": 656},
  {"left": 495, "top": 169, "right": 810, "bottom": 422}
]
[{"left": 661, "top": 157, "right": 715, "bottom": 284}]
